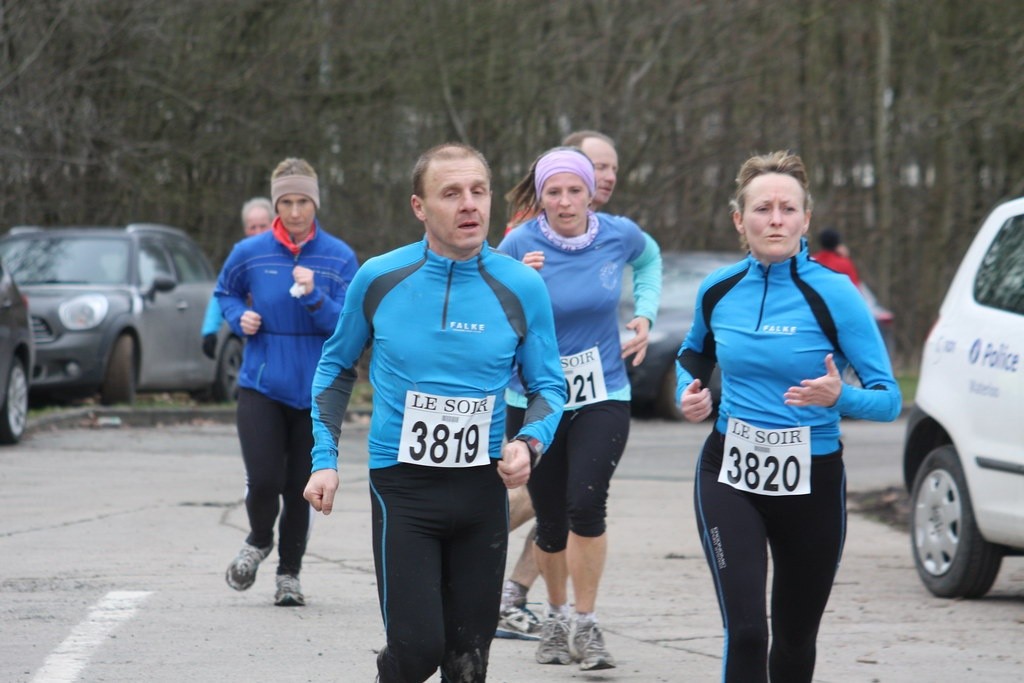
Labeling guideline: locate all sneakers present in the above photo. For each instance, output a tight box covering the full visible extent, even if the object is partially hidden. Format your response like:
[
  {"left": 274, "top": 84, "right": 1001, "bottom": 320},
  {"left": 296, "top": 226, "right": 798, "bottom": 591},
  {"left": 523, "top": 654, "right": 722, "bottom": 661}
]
[
  {"left": 573, "top": 620, "right": 616, "bottom": 670},
  {"left": 535, "top": 613, "right": 572, "bottom": 665},
  {"left": 493, "top": 604, "right": 546, "bottom": 641},
  {"left": 225, "top": 540, "right": 274, "bottom": 591},
  {"left": 275, "top": 570, "right": 305, "bottom": 607}
]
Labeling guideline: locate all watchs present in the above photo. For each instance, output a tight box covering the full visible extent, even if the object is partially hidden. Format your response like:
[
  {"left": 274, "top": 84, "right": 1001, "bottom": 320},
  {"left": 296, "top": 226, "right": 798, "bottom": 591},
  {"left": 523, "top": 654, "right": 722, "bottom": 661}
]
[{"left": 514, "top": 433, "right": 543, "bottom": 470}]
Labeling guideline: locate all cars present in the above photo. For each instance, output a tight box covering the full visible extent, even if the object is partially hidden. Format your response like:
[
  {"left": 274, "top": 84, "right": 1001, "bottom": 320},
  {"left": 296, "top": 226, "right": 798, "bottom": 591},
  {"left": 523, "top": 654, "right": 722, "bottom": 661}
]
[
  {"left": 900, "top": 197, "right": 1023, "bottom": 600},
  {"left": 615, "top": 247, "right": 894, "bottom": 422},
  {"left": 0, "top": 255, "right": 36, "bottom": 448}
]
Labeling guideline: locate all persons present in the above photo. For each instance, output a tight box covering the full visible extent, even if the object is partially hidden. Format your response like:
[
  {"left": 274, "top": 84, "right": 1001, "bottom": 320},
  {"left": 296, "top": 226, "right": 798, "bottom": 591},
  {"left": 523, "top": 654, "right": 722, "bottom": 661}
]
[
  {"left": 811, "top": 229, "right": 859, "bottom": 419},
  {"left": 213, "top": 158, "right": 357, "bottom": 605},
  {"left": 675, "top": 152, "right": 902, "bottom": 682},
  {"left": 495, "top": 132, "right": 662, "bottom": 670},
  {"left": 201, "top": 197, "right": 272, "bottom": 361},
  {"left": 303, "top": 143, "right": 567, "bottom": 683}
]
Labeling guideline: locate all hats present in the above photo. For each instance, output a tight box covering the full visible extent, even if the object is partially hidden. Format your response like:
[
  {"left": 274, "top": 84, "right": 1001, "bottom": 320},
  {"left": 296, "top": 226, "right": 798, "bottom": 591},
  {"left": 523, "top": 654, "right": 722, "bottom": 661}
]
[{"left": 816, "top": 229, "right": 840, "bottom": 253}]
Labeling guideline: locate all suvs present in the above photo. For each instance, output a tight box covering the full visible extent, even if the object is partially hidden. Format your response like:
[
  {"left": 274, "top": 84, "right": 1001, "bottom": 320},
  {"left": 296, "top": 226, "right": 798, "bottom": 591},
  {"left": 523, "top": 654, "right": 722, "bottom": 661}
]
[{"left": 0, "top": 221, "right": 248, "bottom": 408}]
